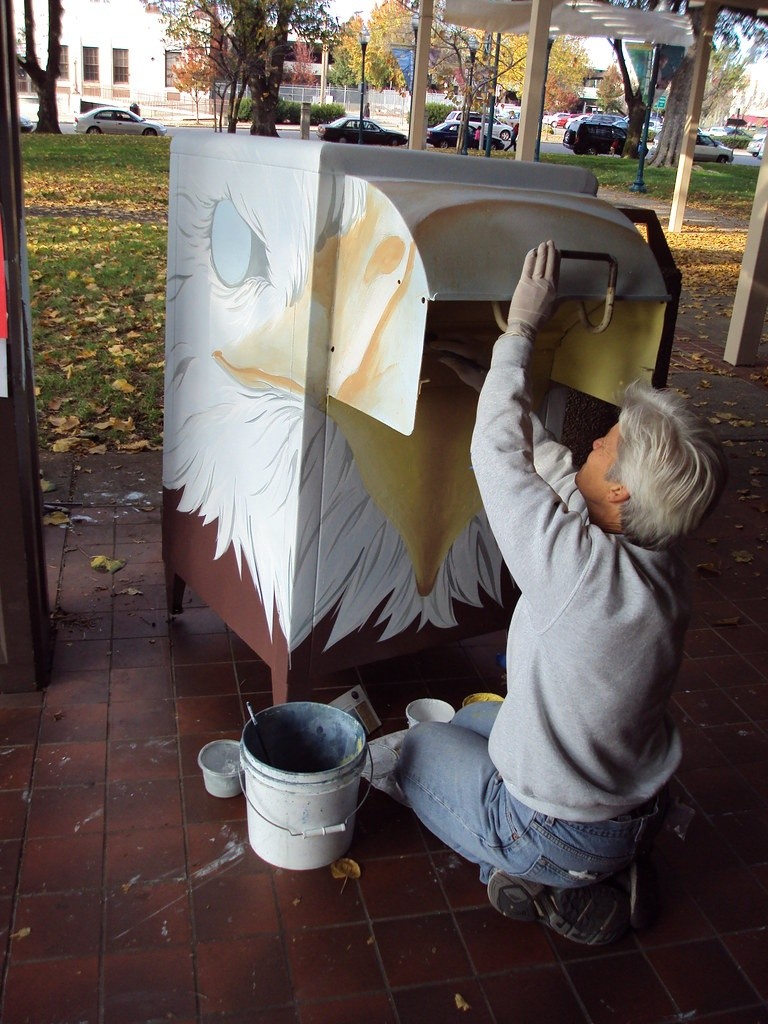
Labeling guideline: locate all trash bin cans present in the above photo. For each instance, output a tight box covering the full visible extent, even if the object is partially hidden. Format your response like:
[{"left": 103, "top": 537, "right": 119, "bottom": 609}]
[
  {"left": 130, "top": 103, "right": 140, "bottom": 116},
  {"left": 238, "top": 701, "right": 374, "bottom": 871}
]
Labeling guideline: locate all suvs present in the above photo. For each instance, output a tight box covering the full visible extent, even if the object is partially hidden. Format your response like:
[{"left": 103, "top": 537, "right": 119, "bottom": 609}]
[{"left": 563, "top": 120, "right": 649, "bottom": 158}]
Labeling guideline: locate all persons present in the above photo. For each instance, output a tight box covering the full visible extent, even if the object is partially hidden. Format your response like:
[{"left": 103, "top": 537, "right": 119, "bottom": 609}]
[
  {"left": 394, "top": 240, "right": 731, "bottom": 950},
  {"left": 609, "top": 139, "right": 619, "bottom": 157},
  {"left": 474, "top": 126, "right": 481, "bottom": 150},
  {"left": 364, "top": 103, "right": 370, "bottom": 119},
  {"left": 504, "top": 123, "right": 519, "bottom": 151}
]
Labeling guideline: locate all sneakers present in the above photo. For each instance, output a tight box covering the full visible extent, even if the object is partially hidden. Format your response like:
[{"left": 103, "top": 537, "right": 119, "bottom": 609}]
[
  {"left": 486, "top": 866, "right": 632, "bottom": 947},
  {"left": 627, "top": 853, "right": 661, "bottom": 930}
]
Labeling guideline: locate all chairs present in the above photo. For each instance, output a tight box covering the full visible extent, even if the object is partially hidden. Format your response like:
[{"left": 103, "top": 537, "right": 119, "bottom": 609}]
[{"left": 353, "top": 123, "right": 357, "bottom": 128}]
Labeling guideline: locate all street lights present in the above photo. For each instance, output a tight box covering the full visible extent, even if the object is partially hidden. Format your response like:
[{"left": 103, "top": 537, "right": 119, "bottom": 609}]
[
  {"left": 462, "top": 36, "right": 479, "bottom": 155},
  {"left": 629, "top": 0, "right": 670, "bottom": 193},
  {"left": 534, "top": 27, "right": 560, "bottom": 163},
  {"left": 357, "top": 30, "right": 371, "bottom": 145},
  {"left": 407, "top": 14, "right": 419, "bottom": 149}
]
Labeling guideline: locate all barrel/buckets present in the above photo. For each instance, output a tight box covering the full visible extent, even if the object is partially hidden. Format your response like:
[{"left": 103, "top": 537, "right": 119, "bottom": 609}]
[
  {"left": 406, "top": 698, "right": 455, "bottom": 730},
  {"left": 236, "top": 702, "right": 373, "bottom": 870},
  {"left": 462, "top": 693, "right": 504, "bottom": 708},
  {"left": 361, "top": 743, "right": 400, "bottom": 797},
  {"left": 198, "top": 738, "right": 245, "bottom": 799}
]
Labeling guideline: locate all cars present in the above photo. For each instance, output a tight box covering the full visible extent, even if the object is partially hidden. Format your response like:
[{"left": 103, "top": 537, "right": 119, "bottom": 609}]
[
  {"left": 654, "top": 132, "right": 734, "bottom": 166},
  {"left": 72, "top": 108, "right": 168, "bottom": 137},
  {"left": 425, "top": 123, "right": 505, "bottom": 151},
  {"left": 542, "top": 112, "right": 767, "bottom": 159},
  {"left": 444, "top": 111, "right": 520, "bottom": 140},
  {"left": 317, "top": 117, "right": 408, "bottom": 146}
]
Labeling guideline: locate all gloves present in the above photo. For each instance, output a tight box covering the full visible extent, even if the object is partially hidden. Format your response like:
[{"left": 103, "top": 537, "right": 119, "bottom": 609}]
[{"left": 497, "top": 240, "right": 564, "bottom": 345}]
